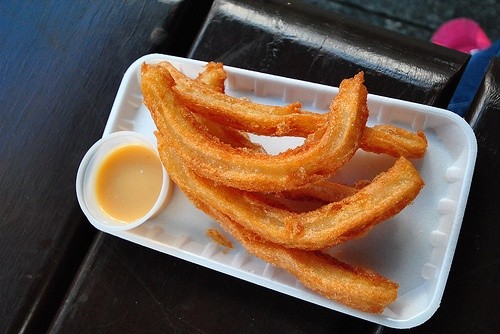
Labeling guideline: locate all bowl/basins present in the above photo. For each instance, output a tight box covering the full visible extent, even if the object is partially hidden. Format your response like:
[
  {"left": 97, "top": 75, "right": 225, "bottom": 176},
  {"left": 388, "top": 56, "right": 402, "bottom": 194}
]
[{"left": 74, "top": 132, "right": 174, "bottom": 231}]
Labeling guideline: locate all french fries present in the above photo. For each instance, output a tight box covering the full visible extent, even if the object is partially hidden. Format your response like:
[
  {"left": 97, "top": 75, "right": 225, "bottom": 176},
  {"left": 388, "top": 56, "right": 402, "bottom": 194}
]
[{"left": 139, "top": 60, "right": 428, "bottom": 312}]
